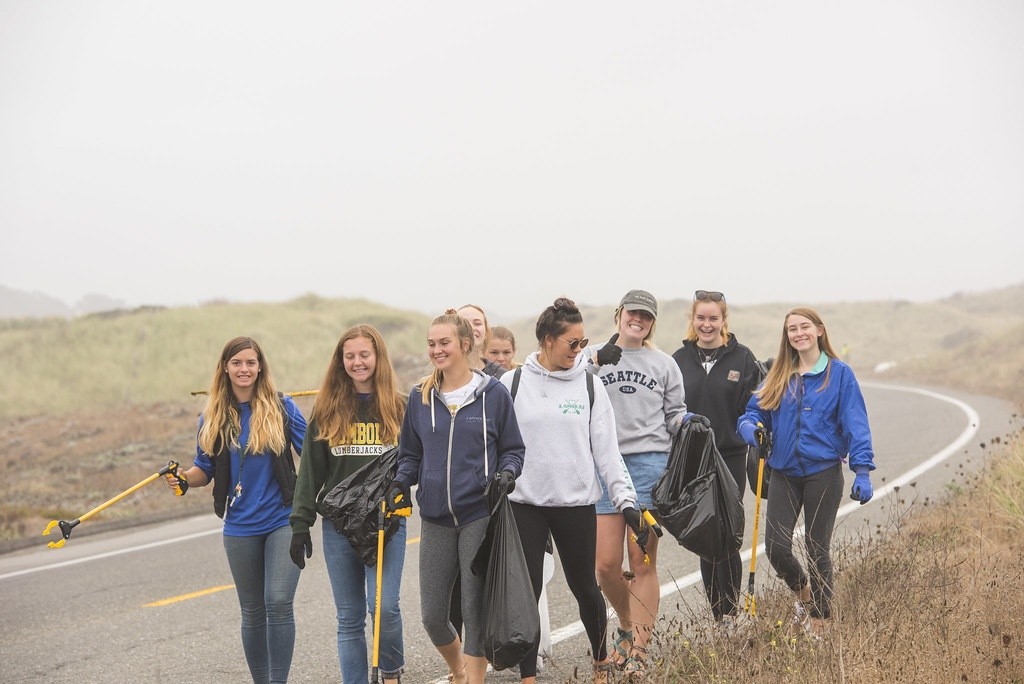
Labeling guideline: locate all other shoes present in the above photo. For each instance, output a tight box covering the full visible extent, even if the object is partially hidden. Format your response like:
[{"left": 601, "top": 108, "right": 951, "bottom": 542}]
[
  {"left": 536, "top": 652, "right": 545, "bottom": 671},
  {"left": 714, "top": 615, "right": 737, "bottom": 632},
  {"left": 793, "top": 602, "right": 830, "bottom": 645},
  {"left": 592, "top": 661, "right": 615, "bottom": 683}
]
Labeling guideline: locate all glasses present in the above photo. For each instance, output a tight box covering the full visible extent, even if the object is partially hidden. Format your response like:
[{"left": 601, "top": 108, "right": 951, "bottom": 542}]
[
  {"left": 693, "top": 290, "right": 727, "bottom": 303},
  {"left": 555, "top": 336, "right": 589, "bottom": 350}
]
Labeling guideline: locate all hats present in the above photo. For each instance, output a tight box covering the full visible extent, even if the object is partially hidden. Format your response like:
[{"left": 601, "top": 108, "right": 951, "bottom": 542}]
[{"left": 619, "top": 289, "right": 657, "bottom": 321}]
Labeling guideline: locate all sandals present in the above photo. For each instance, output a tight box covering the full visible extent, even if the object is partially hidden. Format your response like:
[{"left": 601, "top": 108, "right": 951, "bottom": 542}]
[{"left": 611, "top": 630, "right": 649, "bottom": 677}]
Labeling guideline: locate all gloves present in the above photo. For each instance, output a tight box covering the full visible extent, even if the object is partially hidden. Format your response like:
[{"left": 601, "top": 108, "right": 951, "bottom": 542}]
[
  {"left": 851, "top": 473, "right": 873, "bottom": 504},
  {"left": 385, "top": 481, "right": 404, "bottom": 514},
  {"left": 497, "top": 470, "right": 516, "bottom": 494},
  {"left": 290, "top": 532, "right": 312, "bottom": 569},
  {"left": 623, "top": 506, "right": 649, "bottom": 546},
  {"left": 739, "top": 421, "right": 766, "bottom": 449},
  {"left": 596, "top": 333, "right": 622, "bottom": 366},
  {"left": 683, "top": 412, "right": 712, "bottom": 435}
]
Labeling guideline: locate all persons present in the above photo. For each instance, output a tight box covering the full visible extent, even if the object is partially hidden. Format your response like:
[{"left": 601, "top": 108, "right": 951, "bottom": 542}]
[
  {"left": 499, "top": 295, "right": 650, "bottom": 684},
  {"left": 584, "top": 289, "right": 712, "bottom": 676},
  {"left": 166, "top": 336, "right": 308, "bottom": 684},
  {"left": 384, "top": 308, "right": 525, "bottom": 684},
  {"left": 672, "top": 290, "right": 767, "bottom": 636},
  {"left": 737, "top": 308, "right": 877, "bottom": 641},
  {"left": 457, "top": 304, "right": 551, "bottom": 674},
  {"left": 289, "top": 322, "right": 413, "bottom": 684}
]
